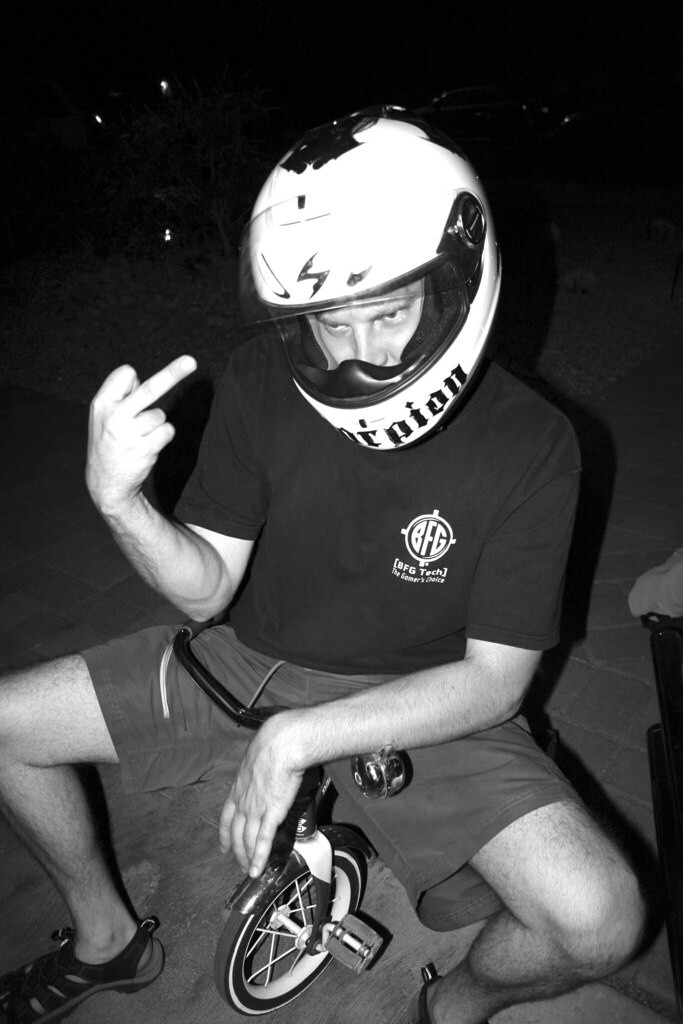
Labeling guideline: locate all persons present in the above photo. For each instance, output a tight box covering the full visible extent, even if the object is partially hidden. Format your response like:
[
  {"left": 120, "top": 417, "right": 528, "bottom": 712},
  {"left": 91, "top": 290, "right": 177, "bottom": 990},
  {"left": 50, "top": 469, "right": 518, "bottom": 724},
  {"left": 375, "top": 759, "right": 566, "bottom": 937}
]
[{"left": 1, "top": 108, "right": 643, "bottom": 1024}]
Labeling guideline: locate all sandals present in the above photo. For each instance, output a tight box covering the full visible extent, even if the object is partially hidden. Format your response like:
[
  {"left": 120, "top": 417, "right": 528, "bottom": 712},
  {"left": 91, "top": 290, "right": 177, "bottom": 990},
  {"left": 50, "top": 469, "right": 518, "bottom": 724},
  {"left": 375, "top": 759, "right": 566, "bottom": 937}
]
[
  {"left": 407, "top": 962, "right": 489, "bottom": 1024},
  {"left": 0, "top": 917, "right": 164, "bottom": 1024}
]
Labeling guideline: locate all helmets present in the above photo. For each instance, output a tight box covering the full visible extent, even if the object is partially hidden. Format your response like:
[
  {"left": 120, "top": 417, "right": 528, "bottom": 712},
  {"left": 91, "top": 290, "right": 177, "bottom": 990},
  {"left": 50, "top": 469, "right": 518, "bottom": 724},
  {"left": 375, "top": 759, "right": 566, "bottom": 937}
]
[{"left": 238, "top": 106, "right": 503, "bottom": 451}]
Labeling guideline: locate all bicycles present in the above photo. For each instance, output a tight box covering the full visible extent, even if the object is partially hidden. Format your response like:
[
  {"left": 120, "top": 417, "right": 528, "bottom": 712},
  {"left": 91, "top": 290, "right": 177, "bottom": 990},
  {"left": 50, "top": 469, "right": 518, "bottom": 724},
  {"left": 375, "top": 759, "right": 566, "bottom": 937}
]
[{"left": 170, "top": 608, "right": 406, "bottom": 1017}]
